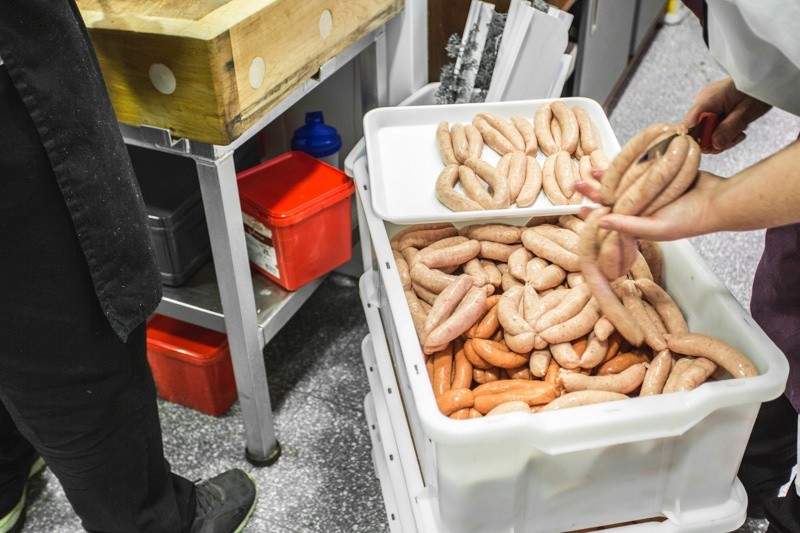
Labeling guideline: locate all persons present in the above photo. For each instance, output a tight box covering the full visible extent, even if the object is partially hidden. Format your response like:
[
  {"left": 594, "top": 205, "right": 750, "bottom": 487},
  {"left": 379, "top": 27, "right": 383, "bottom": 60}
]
[
  {"left": 0, "top": 0, "right": 258, "bottom": 532},
  {"left": 572, "top": 1, "right": 800, "bottom": 533}
]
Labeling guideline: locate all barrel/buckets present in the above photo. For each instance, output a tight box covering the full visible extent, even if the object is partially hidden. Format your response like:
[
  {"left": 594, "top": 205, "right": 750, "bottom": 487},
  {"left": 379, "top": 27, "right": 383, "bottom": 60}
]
[
  {"left": 234, "top": 151, "right": 355, "bottom": 293},
  {"left": 145, "top": 314, "right": 239, "bottom": 418}
]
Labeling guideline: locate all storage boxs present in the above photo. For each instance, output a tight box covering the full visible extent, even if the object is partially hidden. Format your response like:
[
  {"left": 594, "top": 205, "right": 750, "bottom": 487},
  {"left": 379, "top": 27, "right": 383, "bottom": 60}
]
[
  {"left": 144, "top": 314, "right": 237, "bottom": 418},
  {"left": 79, "top": 0, "right": 410, "bottom": 145},
  {"left": 237, "top": 148, "right": 354, "bottom": 294},
  {"left": 350, "top": 149, "right": 791, "bottom": 533}
]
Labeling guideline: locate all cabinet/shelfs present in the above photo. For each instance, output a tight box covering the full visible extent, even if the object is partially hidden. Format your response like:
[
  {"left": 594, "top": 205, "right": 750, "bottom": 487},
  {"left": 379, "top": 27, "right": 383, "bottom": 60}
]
[{"left": 93, "top": 21, "right": 404, "bottom": 467}]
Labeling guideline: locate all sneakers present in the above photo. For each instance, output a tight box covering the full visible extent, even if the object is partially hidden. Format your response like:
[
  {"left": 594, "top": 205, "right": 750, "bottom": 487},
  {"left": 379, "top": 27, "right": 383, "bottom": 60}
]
[
  {"left": 0, "top": 440, "right": 47, "bottom": 533},
  {"left": 182, "top": 467, "right": 258, "bottom": 533}
]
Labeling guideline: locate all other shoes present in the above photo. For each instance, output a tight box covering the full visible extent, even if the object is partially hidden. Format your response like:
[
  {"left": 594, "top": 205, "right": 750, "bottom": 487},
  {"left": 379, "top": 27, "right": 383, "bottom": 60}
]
[{"left": 746, "top": 489, "right": 777, "bottom": 520}]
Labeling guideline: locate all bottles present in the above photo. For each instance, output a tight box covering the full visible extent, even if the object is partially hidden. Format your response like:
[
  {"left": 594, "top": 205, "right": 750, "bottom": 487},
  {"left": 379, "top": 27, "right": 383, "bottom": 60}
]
[{"left": 289, "top": 111, "right": 342, "bottom": 169}]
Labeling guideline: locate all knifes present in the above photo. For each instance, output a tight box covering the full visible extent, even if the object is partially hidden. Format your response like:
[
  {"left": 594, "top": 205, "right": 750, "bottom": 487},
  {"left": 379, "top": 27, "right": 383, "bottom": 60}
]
[{"left": 635, "top": 110, "right": 720, "bottom": 165}]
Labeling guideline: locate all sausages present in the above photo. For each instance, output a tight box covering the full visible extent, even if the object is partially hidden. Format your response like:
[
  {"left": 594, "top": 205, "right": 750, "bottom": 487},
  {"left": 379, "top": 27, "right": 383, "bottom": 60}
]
[
  {"left": 435, "top": 100, "right": 613, "bottom": 213},
  {"left": 388, "top": 209, "right": 758, "bottom": 421},
  {"left": 599, "top": 121, "right": 701, "bottom": 218}
]
